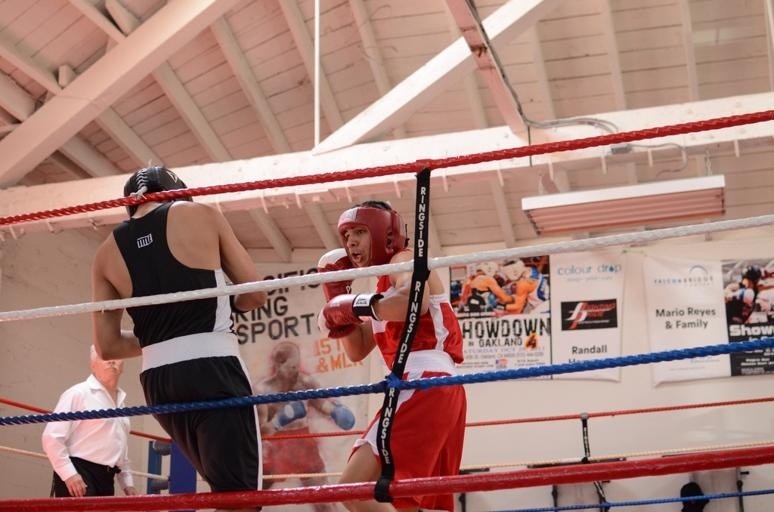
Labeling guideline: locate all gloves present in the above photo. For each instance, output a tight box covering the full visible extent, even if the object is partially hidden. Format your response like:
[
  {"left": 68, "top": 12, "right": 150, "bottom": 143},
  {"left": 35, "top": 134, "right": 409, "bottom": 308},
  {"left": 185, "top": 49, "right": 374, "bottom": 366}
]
[
  {"left": 318, "top": 293, "right": 383, "bottom": 338},
  {"left": 273, "top": 401, "right": 306, "bottom": 428},
  {"left": 488, "top": 293, "right": 498, "bottom": 308},
  {"left": 331, "top": 403, "right": 355, "bottom": 430},
  {"left": 732, "top": 287, "right": 754, "bottom": 304},
  {"left": 511, "top": 284, "right": 516, "bottom": 294},
  {"left": 318, "top": 248, "right": 355, "bottom": 301}
]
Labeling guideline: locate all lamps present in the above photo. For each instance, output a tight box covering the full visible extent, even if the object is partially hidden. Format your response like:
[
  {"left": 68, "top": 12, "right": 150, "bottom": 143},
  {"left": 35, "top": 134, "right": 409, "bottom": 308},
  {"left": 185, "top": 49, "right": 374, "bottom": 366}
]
[{"left": 313, "top": 0, "right": 548, "bottom": 152}]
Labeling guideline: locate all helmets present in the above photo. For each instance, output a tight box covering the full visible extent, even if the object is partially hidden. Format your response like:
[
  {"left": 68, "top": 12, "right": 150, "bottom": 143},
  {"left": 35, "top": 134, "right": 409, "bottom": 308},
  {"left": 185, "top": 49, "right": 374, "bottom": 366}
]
[
  {"left": 477, "top": 262, "right": 499, "bottom": 277},
  {"left": 499, "top": 260, "right": 525, "bottom": 281},
  {"left": 338, "top": 207, "right": 406, "bottom": 267},
  {"left": 124, "top": 167, "right": 193, "bottom": 216},
  {"left": 742, "top": 267, "right": 761, "bottom": 280}
]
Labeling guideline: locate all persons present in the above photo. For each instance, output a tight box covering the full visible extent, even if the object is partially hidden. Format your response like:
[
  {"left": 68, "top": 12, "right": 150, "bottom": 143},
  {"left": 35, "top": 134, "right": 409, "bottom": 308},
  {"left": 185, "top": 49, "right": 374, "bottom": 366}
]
[
  {"left": 726, "top": 265, "right": 764, "bottom": 325},
  {"left": 40, "top": 343, "right": 140, "bottom": 497},
  {"left": 316, "top": 201, "right": 470, "bottom": 511},
  {"left": 89, "top": 164, "right": 269, "bottom": 512},
  {"left": 252, "top": 340, "right": 356, "bottom": 510},
  {"left": 451, "top": 259, "right": 549, "bottom": 313}
]
